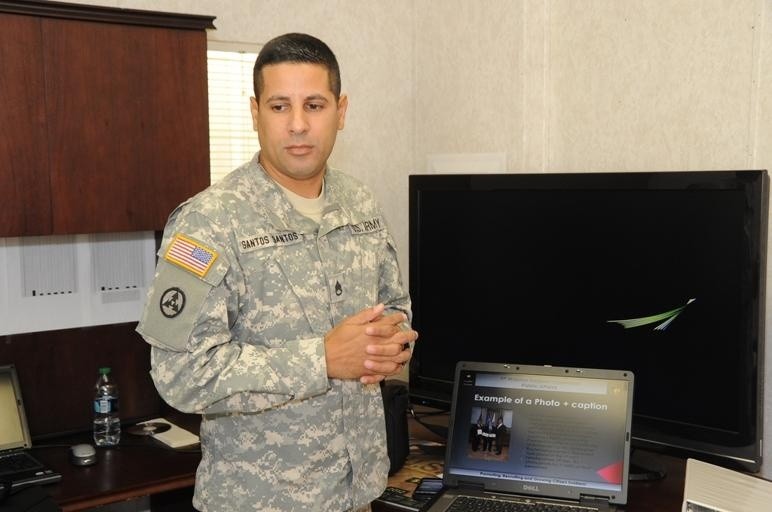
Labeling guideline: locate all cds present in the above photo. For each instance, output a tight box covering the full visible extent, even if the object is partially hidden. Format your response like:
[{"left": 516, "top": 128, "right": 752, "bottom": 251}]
[{"left": 126, "top": 422, "right": 170, "bottom": 435}]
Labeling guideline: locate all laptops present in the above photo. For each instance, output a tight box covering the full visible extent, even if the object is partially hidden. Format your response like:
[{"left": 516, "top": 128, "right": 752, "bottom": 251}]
[
  {"left": 419, "top": 361, "right": 634, "bottom": 512},
  {"left": 0, "top": 363, "right": 61, "bottom": 490}
]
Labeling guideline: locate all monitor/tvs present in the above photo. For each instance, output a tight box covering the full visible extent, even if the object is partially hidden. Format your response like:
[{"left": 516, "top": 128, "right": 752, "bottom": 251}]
[{"left": 409, "top": 171, "right": 770, "bottom": 473}]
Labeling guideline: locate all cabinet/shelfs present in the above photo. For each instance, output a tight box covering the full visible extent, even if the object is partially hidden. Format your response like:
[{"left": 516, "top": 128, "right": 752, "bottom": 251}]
[{"left": 1, "top": 1, "right": 210, "bottom": 234}]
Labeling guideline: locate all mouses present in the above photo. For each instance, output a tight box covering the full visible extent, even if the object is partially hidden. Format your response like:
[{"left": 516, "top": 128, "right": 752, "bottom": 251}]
[{"left": 69, "top": 443, "right": 98, "bottom": 466}]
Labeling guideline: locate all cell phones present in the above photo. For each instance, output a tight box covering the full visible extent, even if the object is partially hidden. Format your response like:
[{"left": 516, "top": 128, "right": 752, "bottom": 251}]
[{"left": 413, "top": 477, "right": 443, "bottom": 500}]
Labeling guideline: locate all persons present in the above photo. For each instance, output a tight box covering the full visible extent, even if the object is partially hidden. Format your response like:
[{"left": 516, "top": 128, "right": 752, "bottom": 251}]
[
  {"left": 471, "top": 413, "right": 507, "bottom": 454},
  {"left": 135, "top": 34, "right": 419, "bottom": 512}
]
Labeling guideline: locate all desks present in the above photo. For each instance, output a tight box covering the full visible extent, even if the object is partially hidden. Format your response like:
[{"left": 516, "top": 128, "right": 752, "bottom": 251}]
[
  {"left": 1, "top": 408, "right": 203, "bottom": 512},
  {"left": 372, "top": 403, "right": 683, "bottom": 509}
]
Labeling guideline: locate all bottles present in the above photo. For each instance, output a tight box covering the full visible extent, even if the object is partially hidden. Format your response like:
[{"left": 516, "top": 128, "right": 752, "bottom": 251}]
[{"left": 91, "top": 367, "right": 120, "bottom": 448}]
